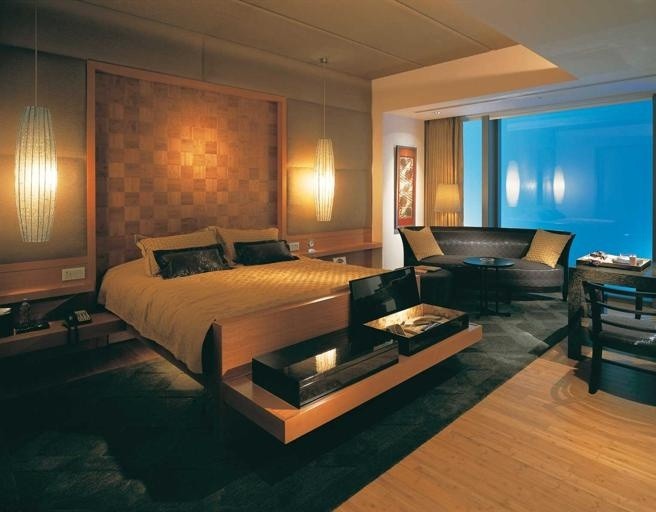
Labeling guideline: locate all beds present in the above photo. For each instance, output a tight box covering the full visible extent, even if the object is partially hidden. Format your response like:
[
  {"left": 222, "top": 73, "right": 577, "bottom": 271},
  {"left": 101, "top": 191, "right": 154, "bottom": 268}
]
[{"left": 99, "top": 224, "right": 399, "bottom": 375}]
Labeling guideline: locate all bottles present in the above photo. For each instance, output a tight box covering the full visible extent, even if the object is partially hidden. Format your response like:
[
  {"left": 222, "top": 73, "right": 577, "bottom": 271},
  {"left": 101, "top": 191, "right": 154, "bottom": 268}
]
[{"left": 19, "top": 300, "right": 31, "bottom": 328}]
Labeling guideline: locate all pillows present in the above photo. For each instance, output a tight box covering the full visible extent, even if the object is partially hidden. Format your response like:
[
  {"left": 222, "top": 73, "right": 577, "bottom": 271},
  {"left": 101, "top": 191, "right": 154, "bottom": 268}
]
[
  {"left": 399, "top": 228, "right": 445, "bottom": 261},
  {"left": 150, "top": 244, "right": 234, "bottom": 280},
  {"left": 133, "top": 225, "right": 231, "bottom": 279},
  {"left": 522, "top": 229, "right": 573, "bottom": 269},
  {"left": 216, "top": 225, "right": 280, "bottom": 266},
  {"left": 233, "top": 240, "right": 301, "bottom": 265}
]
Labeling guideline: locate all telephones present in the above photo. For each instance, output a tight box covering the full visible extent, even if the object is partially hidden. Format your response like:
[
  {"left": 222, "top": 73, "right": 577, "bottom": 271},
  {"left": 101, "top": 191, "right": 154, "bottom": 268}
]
[{"left": 65, "top": 309, "right": 91, "bottom": 326}]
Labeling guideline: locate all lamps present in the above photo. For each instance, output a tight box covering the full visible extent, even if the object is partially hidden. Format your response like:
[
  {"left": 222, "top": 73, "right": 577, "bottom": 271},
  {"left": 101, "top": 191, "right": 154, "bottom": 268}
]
[
  {"left": 313, "top": 57, "right": 336, "bottom": 222},
  {"left": 15, "top": 0, "right": 60, "bottom": 243}
]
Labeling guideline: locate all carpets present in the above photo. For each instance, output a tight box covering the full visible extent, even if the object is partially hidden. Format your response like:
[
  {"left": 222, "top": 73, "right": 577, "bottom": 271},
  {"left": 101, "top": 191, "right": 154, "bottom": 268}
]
[{"left": 1, "top": 271, "right": 572, "bottom": 512}]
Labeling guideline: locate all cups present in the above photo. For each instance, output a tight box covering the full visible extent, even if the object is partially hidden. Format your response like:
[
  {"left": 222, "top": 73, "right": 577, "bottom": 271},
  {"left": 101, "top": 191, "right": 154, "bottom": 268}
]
[
  {"left": 385, "top": 324, "right": 406, "bottom": 336},
  {"left": 630, "top": 255, "right": 638, "bottom": 267}
]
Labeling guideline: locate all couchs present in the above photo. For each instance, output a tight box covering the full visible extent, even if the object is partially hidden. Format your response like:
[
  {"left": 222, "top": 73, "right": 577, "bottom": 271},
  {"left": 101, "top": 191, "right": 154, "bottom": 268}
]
[{"left": 398, "top": 226, "right": 576, "bottom": 302}]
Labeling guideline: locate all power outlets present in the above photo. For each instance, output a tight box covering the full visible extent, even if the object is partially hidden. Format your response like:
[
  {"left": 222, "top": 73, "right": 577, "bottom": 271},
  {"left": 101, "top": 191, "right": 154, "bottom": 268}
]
[
  {"left": 60, "top": 267, "right": 85, "bottom": 281},
  {"left": 289, "top": 242, "right": 300, "bottom": 252}
]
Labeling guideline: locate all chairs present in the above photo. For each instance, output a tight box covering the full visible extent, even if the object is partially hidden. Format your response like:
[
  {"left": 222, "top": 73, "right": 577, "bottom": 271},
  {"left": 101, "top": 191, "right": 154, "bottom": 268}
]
[{"left": 585, "top": 279, "right": 656, "bottom": 394}]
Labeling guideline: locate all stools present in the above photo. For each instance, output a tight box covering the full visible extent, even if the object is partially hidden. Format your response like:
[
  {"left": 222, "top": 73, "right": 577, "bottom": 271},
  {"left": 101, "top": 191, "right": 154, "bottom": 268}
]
[{"left": 394, "top": 265, "right": 453, "bottom": 311}]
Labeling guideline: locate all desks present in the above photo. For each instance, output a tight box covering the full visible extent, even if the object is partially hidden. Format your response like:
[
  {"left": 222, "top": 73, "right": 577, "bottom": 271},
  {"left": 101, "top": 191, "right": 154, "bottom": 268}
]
[{"left": 566, "top": 251, "right": 656, "bottom": 360}]
